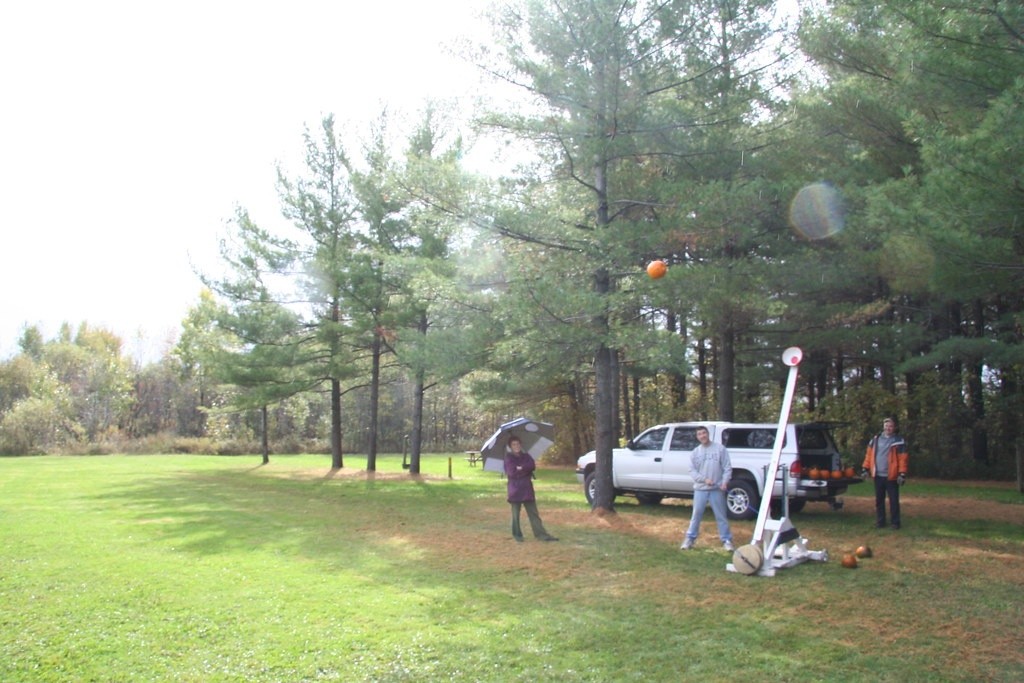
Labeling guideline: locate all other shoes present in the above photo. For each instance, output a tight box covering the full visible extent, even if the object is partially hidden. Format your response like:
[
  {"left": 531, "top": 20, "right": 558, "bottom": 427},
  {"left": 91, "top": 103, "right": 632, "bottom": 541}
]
[
  {"left": 513, "top": 534, "right": 524, "bottom": 542},
  {"left": 873, "top": 522, "right": 886, "bottom": 528},
  {"left": 724, "top": 541, "right": 734, "bottom": 551},
  {"left": 680, "top": 537, "right": 694, "bottom": 549},
  {"left": 890, "top": 523, "right": 900, "bottom": 529},
  {"left": 544, "top": 535, "right": 559, "bottom": 541}
]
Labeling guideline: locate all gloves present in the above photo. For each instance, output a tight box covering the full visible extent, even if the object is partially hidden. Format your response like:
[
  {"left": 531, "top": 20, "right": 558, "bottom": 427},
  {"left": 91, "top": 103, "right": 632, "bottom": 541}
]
[
  {"left": 860, "top": 468, "right": 869, "bottom": 481},
  {"left": 896, "top": 473, "right": 906, "bottom": 486}
]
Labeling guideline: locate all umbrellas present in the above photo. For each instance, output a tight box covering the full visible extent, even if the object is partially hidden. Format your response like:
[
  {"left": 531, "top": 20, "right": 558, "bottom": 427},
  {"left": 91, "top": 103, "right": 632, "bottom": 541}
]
[{"left": 481, "top": 417, "right": 554, "bottom": 481}]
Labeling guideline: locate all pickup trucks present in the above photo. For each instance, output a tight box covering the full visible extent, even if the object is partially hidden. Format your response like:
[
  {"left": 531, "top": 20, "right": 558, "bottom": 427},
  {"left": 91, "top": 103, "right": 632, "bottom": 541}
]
[{"left": 576, "top": 419, "right": 853, "bottom": 520}]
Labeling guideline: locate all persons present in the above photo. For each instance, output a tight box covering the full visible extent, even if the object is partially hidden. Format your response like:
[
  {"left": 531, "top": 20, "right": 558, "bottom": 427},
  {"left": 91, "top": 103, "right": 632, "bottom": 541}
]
[
  {"left": 861, "top": 419, "right": 909, "bottom": 528},
  {"left": 680, "top": 426, "right": 734, "bottom": 552},
  {"left": 504, "top": 437, "right": 557, "bottom": 540}
]
